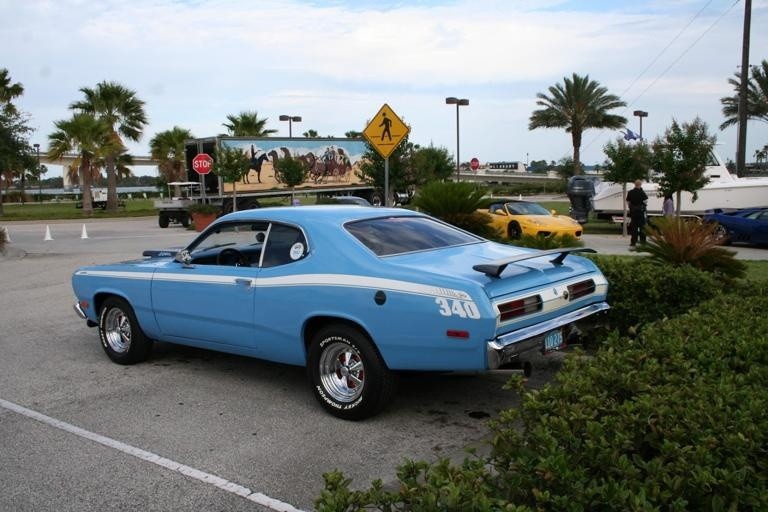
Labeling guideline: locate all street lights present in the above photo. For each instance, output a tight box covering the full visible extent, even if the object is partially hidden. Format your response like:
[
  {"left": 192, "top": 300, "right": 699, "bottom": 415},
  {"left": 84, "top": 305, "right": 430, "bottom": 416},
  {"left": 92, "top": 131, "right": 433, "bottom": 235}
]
[
  {"left": 278, "top": 115, "right": 302, "bottom": 206},
  {"left": 445, "top": 98, "right": 470, "bottom": 183},
  {"left": 34, "top": 144, "right": 42, "bottom": 203},
  {"left": 633, "top": 111, "right": 649, "bottom": 143}
]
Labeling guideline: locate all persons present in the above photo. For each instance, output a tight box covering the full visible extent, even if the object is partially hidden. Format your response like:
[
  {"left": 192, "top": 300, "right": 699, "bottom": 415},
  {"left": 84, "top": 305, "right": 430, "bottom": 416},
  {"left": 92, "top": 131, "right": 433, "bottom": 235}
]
[
  {"left": 660, "top": 191, "right": 675, "bottom": 220},
  {"left": 623, "top": 179, "right": 651, "bottom": 249}
]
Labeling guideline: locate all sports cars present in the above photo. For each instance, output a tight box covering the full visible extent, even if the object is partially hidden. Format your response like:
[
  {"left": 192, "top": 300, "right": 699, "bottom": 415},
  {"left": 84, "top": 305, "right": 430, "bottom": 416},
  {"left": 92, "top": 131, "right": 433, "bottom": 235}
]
[{"left": 476, "top": 201, "right": 584, "bottom": 244}]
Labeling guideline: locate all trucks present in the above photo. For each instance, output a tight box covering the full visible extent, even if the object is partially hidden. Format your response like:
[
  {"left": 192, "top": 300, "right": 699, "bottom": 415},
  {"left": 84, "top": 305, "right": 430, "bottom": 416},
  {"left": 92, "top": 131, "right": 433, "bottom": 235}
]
[{"left": 184, "top": 133, "right": 420, "bottom": 216}]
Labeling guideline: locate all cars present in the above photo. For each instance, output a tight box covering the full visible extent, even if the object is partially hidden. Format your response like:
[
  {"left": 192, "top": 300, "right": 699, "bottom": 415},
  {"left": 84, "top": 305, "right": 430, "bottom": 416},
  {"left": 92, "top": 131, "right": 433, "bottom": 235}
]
[
  {"left": 71, "top": 203, "right": 611, "bottom": 424},
  {"left": 700, "top": 206, "right": 768, "bottom": 246}
]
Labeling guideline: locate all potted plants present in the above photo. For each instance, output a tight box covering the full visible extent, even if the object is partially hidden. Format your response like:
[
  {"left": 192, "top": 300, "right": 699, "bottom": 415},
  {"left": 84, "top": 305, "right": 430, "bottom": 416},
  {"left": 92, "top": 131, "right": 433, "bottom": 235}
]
[{"left": 186, "top": 205, "right": 223, "bottom": 231}]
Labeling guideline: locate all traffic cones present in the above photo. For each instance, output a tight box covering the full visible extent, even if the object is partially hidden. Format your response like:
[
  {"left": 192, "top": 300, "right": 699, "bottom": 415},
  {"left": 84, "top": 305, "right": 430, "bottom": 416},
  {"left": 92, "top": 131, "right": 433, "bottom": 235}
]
[
  {"left": 80, "top": 224, "right": 89, "bottom": 239},
  {"left": 5, "top": 226, "right": 10, "bottom": 242},
  {"left": 43, "top": 224, "right": 53, "bottom": 241}
]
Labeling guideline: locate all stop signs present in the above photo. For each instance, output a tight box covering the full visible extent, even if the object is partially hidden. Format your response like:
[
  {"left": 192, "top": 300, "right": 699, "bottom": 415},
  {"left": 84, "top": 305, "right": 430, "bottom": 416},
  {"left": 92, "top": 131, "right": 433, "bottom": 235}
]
[
  {"left": 193, "top": 154, "right": 214, "bottom": 174},
  {"left": 470, "top": 158, "right": 479, "bottom": 170}
]
